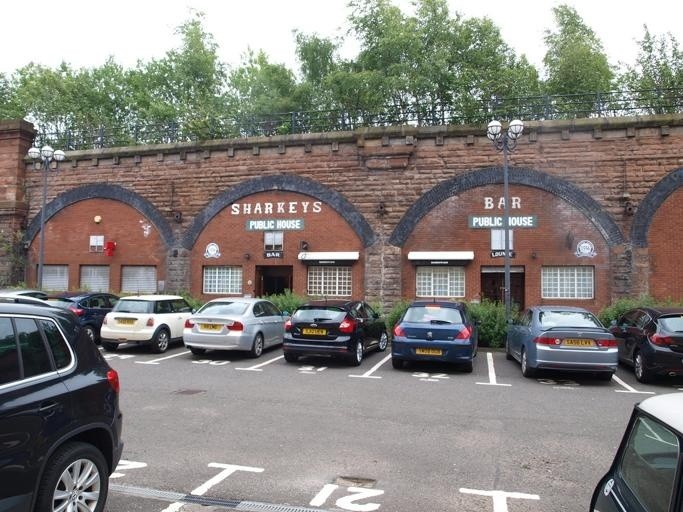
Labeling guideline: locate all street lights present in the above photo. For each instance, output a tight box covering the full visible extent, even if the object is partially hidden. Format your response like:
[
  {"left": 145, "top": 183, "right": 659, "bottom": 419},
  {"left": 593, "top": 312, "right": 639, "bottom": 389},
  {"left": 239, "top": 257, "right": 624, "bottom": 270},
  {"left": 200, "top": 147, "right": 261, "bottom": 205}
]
[
  {"left": 484, "top": 117, "right": 527, "bottom": 328},
  {"left": 27, "top": 147, "right": 67, "bottom": 287}
]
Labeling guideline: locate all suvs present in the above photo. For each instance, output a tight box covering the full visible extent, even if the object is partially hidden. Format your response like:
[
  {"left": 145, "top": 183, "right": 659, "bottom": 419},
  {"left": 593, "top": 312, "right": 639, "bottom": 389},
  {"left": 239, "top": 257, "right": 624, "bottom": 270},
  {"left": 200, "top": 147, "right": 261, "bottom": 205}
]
[{"left": 0, "top": 291, "right": 124, "bottom": 512}]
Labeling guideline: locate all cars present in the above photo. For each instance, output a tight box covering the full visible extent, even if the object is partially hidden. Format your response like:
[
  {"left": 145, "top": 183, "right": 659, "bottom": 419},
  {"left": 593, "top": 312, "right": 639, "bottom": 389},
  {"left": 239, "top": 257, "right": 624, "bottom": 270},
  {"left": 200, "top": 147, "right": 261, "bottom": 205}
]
[
  {"left": 283, "top": 296, "right": 390, "bottom": 366},
  {"left": 53, "top": 293, "right": 122, "bottom": 349},
  {"left": 609, "top": 306, "right": 682, "bottom": 386},
  {"left": 0, "top": 288, "right": 48, "bottom": 303},
  {"left": 180, "top": 297, "right": 288, "bottom": 356},
  {"left": 504, "top": 306, "right": 619, "bottom": 383},
  {"left": 390, "top": 297, "right": 478, "bottom": 372},
  {"left": 100, "top": 292, "right": 197, "bottom": 356}
]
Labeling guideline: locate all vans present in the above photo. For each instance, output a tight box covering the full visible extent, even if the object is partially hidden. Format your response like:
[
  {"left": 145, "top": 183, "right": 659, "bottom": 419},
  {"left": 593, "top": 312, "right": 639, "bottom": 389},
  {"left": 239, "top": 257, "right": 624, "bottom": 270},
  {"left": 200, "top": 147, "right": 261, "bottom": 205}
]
[{"left": 591, "top": 391, "right": 682, "bottom": 510}]
